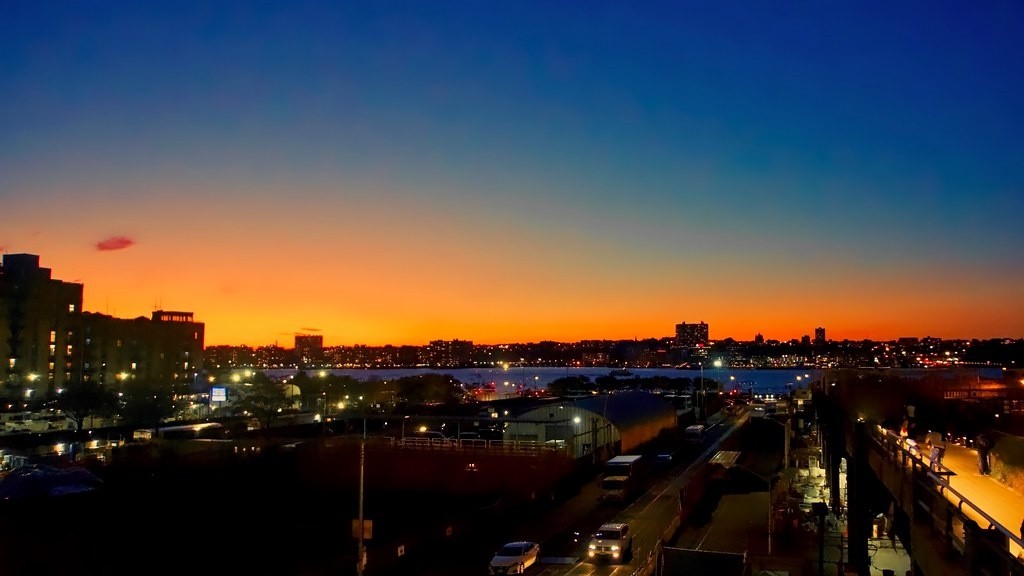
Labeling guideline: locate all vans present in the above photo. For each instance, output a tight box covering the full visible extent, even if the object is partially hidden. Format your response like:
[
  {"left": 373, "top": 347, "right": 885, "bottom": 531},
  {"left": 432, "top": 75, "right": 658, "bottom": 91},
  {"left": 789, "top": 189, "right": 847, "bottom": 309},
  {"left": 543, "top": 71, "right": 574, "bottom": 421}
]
[{"left": 685, "top": 425, "right": 706, "bottom": 446}]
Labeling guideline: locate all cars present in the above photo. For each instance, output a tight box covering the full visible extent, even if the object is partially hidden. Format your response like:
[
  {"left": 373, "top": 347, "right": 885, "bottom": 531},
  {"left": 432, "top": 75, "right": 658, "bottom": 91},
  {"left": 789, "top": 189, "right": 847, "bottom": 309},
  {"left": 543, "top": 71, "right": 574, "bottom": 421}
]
[
  {"left": 656, "top": 454, "right": 671, "bottom": 465},
  {"left": 488, "top": 541, "right": 540, "bottom": 575}
]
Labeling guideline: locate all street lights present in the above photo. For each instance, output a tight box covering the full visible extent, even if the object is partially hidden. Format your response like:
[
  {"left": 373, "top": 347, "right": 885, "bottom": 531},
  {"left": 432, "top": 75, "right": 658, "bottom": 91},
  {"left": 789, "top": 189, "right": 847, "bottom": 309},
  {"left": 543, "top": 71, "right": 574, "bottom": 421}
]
[
  {"left": 762, "top": 417, "right": 788, "bottom": 469},
  {"left": 722, "top": 462, "right": 772, "bottom": 556}
]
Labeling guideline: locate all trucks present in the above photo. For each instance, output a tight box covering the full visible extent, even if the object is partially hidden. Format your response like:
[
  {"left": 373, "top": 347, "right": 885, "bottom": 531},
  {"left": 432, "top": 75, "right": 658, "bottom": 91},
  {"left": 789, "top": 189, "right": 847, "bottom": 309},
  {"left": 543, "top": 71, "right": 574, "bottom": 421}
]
[{"left": 601, "top": 455, "right": 642, "bottom": 503}]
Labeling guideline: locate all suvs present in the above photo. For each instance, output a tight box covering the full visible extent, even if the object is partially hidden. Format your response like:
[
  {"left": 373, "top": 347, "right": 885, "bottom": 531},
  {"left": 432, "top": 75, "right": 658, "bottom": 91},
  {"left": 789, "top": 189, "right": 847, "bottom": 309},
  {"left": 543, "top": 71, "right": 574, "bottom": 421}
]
[{"left": 587, "top": 523, "right": 631, "bottom": 562}]
